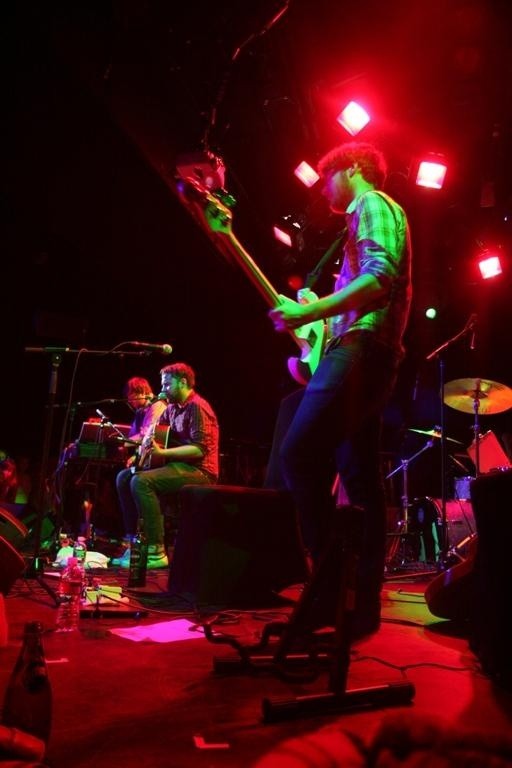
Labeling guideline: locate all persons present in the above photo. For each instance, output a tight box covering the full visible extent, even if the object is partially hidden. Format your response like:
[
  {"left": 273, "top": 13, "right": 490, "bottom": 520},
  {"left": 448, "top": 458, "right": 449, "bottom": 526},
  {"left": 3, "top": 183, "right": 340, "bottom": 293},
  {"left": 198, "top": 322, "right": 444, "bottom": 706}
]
[
  {"left": 264, "top": 142, "right": 413, "bottom": 649},
  {"left": 111, "top": 360, "right": 223, "bottom": 571},
  {"left": 121, "top": 373, "right": 168, "bottom": 545}
]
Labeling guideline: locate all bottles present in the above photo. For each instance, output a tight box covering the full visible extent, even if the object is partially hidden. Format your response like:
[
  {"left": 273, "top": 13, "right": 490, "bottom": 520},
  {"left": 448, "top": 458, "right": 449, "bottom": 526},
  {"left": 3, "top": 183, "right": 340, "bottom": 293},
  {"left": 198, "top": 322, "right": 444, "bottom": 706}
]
[
  {"left": 74, "top": 537, "right": 87, "bottom": 582},
  {"left": 56, "top": 558, "right": 84, "bottom": 633},
  {"left": 126, "top": 517, "right": 151, "bottom": 589}
]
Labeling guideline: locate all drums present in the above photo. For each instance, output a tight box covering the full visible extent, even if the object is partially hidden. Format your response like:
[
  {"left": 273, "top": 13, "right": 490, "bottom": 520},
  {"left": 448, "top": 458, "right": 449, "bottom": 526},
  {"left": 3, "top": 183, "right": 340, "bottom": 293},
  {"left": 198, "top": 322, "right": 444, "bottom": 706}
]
[
  {"left": 401, "top": 495, "right": 479, "bottom": 571},
  {"left": 454, "top": 476, "right": 475, "bottom": 500}
]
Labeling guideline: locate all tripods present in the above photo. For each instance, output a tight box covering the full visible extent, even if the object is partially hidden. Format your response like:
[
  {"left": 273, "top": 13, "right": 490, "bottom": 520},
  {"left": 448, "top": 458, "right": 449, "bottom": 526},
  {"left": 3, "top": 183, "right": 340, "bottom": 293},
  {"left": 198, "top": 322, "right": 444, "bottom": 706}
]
[
  {"left": 20, "top": 479, "right": 62, "bottom": 608},
  {"left": 383, "top": 361, "right": 445, "bottom": 582}
]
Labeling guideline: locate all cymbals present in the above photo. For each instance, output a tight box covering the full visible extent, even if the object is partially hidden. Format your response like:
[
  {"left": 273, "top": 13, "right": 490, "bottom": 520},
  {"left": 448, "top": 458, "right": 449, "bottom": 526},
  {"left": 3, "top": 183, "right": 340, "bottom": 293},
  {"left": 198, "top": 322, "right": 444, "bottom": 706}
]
[
  {"left": 409, "top": 428, "right": 466, "bottom": 448},
  {"left": 438, "top": 378, "right": 512, "bottom": 414}
]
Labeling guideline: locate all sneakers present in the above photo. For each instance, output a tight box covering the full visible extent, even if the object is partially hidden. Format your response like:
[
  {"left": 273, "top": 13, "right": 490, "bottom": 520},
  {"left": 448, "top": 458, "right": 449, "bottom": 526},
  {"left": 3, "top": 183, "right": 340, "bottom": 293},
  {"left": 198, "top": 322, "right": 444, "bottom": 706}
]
[
  {"left": 287, "top": 574, "right": 382, "bottom": 628},
  {"left": 112, "top": 544, "right": 169, "bottom": 569}
]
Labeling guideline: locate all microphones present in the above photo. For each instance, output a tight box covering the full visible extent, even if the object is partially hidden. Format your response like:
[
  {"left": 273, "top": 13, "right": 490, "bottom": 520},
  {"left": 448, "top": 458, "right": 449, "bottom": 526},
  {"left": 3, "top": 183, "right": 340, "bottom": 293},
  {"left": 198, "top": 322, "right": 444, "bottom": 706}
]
[
  {"left": 117, "top": 437, "right": 142, "bottom": 446},
  {"left": 467, "top": 313, "right": 480, "bottom": 350},
  {"left": 128, "top": 341, "right": 173, "bottom": 355},
  {"left": 157, "top": 393, "right": 167, "bottom": 401}
]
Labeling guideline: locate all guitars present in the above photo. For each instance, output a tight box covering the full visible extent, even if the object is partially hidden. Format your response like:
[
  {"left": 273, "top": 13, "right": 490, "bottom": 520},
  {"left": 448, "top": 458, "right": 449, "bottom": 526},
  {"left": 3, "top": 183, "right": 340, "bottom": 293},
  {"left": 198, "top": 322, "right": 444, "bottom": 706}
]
[
  {"left": 205, "top": 187, "right": 328, "bottom": 388},
  {"left": 144, "top": 425, "right": 171, "bottom": 471}
]
